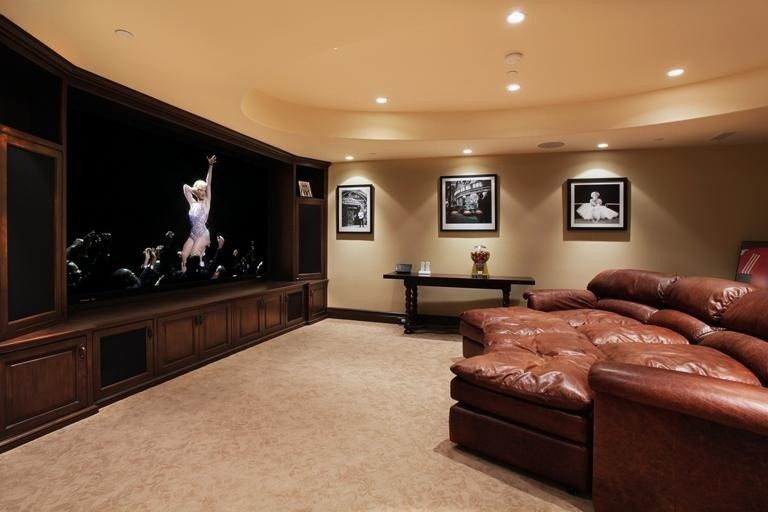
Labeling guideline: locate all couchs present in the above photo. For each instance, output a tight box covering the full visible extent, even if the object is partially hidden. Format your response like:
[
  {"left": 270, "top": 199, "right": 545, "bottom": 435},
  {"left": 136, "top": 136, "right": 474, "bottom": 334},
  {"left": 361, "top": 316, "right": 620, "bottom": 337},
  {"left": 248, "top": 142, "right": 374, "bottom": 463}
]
[{"left": 525, "top": 265, "right": 768, "bottom": 511}]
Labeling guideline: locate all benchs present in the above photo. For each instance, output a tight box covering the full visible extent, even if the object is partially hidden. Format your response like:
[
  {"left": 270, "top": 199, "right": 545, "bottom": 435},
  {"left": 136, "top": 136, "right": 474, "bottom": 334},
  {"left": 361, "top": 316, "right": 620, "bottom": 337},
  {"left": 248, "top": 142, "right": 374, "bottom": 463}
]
[{"left": 449, "top": 305, "right": 598, "bottom": 496}]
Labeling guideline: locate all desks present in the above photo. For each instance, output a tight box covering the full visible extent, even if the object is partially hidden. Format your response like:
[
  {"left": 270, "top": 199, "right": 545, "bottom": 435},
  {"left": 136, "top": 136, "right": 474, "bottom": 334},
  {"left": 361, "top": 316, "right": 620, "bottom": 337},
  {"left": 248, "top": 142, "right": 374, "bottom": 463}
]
[{"left": 383, "top": 272, "right": 536, "bottom": 334}]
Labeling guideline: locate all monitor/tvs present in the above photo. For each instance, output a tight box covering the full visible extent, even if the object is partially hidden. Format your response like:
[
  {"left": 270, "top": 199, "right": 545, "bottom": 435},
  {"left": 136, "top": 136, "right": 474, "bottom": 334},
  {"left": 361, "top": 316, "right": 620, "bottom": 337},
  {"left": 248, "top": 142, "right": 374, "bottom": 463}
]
[{"left": 67, "top": 90, "right": 269, "bottom": 310}]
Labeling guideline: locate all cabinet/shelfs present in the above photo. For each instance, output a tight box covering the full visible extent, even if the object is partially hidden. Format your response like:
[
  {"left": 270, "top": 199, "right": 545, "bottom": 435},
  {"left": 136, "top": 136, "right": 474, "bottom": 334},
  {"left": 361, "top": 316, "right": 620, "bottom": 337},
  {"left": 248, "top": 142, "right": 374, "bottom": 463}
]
[
  {"left": 0, "top": 53, "right": 70, "bottom": 342},
  {"left": 269, "top": 157, "right": 331, "bottom": 281},
  {"left": 0, "top": 278, "right": 328, "bottom": 453}
]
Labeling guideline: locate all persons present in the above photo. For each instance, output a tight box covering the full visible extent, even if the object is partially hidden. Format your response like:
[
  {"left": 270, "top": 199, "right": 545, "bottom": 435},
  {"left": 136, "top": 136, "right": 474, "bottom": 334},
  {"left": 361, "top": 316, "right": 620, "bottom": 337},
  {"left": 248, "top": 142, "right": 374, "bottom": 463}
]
[
  {"left": 181, "top": 154, "right": 217, "bottom": 271},
  {"left": 358, "top": 209, "right": 364, "bottom": 227},
  {"left": 575, "top": 191, "right": 619, "bottom": 224},
  {"left": 66, "top": 229, "right": 265, "bottom": 294}
]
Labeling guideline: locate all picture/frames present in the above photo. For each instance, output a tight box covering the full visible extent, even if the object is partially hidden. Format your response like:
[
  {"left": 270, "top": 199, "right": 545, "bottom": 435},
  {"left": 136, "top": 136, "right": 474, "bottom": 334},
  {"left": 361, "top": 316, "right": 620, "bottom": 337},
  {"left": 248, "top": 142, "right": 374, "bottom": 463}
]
[
  {"left": 566, "top": 177, "right": 628, "bottom": 232},
  {"left": 439, "top": 174, "right": 498, "bottom": 233},
  {"left": 335, "top": 185, "right": 372, "bottom": 235}
]
